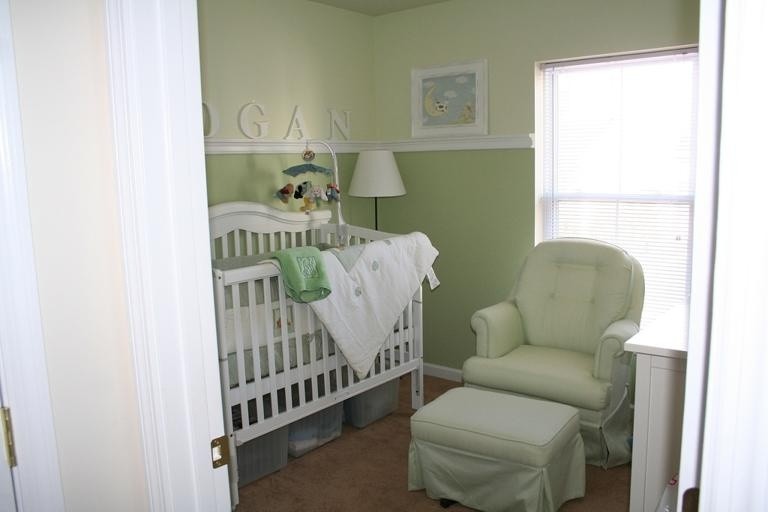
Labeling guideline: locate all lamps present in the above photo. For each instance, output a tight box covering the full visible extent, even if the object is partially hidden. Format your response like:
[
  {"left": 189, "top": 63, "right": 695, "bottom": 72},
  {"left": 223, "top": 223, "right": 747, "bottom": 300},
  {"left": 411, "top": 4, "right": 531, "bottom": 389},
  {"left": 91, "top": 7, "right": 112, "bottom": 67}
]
[{"left": 348, "top": 149, "right": 407, "bottom": 230}]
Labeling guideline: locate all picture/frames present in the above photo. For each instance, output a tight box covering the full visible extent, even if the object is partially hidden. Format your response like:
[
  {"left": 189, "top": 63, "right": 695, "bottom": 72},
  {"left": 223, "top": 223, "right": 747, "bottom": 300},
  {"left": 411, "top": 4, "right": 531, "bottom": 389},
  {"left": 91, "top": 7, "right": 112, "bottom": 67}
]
[{"left": 411, "top": 57, "right": 487, "bottom": 140}]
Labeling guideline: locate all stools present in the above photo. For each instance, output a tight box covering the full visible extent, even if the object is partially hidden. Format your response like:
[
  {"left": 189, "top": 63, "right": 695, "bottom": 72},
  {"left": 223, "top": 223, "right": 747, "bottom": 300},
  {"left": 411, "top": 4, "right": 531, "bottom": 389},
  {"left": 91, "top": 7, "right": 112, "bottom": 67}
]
[{"left": 407, "top": 386, "right": 585, "bottom": 511}]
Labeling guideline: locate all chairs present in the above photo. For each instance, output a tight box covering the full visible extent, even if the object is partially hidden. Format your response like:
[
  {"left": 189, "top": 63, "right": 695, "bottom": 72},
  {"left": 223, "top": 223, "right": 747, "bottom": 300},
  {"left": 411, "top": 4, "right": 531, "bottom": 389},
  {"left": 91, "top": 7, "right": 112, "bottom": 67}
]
[{"left": 458, "top": 235, "right": 645, "bottom": 470}]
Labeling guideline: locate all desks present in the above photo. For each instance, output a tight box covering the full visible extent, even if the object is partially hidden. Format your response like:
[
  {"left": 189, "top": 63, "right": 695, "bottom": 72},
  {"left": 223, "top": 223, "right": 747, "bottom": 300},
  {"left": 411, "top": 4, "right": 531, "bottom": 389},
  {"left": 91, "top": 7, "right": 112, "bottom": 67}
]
[{"left": 624, "top": 319, "right": 688, "bottom": 512}]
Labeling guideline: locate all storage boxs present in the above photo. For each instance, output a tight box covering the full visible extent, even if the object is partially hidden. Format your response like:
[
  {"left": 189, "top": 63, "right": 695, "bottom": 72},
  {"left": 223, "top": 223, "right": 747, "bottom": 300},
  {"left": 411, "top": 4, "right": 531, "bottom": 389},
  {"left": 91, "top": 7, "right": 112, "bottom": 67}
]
[{"left": 232, "top": 364, "right": 402, "bottom": 489}]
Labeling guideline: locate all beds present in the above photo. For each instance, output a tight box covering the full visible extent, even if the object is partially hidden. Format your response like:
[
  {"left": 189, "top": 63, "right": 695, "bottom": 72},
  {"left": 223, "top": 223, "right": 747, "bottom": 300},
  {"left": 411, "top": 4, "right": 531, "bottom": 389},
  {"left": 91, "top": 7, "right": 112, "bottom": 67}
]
[{"left": 208, "top": 200, "right": 424, "bottom": 444}]
